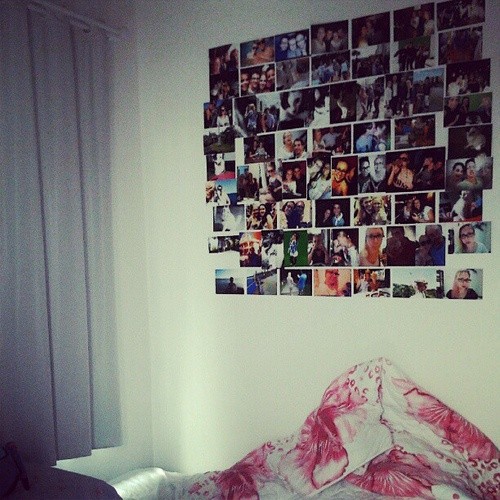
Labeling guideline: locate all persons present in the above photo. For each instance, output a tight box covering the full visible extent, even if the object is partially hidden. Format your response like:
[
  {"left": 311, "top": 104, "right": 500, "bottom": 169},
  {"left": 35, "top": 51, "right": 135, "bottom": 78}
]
[{"left": 203, "top": 0, "right": 495, "bottom": 301}]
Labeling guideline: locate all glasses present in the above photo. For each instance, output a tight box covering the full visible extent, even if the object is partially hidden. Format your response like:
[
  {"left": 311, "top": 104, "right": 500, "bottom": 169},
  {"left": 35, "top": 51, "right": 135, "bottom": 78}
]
[
  {"left": 374, "top": 161, "right": 386, "bottom": 166},
  {"left": 295, "top": 37, "right": 305, "bottom": 43},
  {"left": 323, "top": 167, "right": 330, "bottom": 170},
  {"left": 315, "top": 162, "right": 321, "bottom": 169},
  {"left": 252, "top": 46, "right": 259, "bottom": 50},
  {"left": 285, "top": 135, "right": 293, "bottom": 138},
  {"left": 267, "top": 170, "right": 274, "bottom": 173},
  {"left": 297, "top": 205, "right": 305, "bottom": 209},
  {"left": 457, "top": 277, "right": 471, "bottom": 283},
  {"left": 361, "top": 166, "right": 370, "bottom": 171},
  {"left": 220, "top": 109, "right": 225, "bottom": 112},
  {"left": 218, "top": 189, "right": 223, "bottom": 192},
  {"left": 399, "top": 156, "right": 409, "bottom": 163},
  {"left": 281, "top": 41, "right": 288, "bottom": 45},
  {"left": 366, "top": 233, "right": 385, "bottom": 240},
  {"left": 287, "top": 205, "right": 294, "bottom": 211},
  {"left": 335, "top": 169, "right": 347, "bottom": 175},
  {"left": 290, "top": 43, "right": 296, "bottom": 47},
  {"left": 326, "top": 271, "right": 341, "bottom": 277},
  {"left": 460, "top": 232, "right": 475, "bottom": 240}
]
